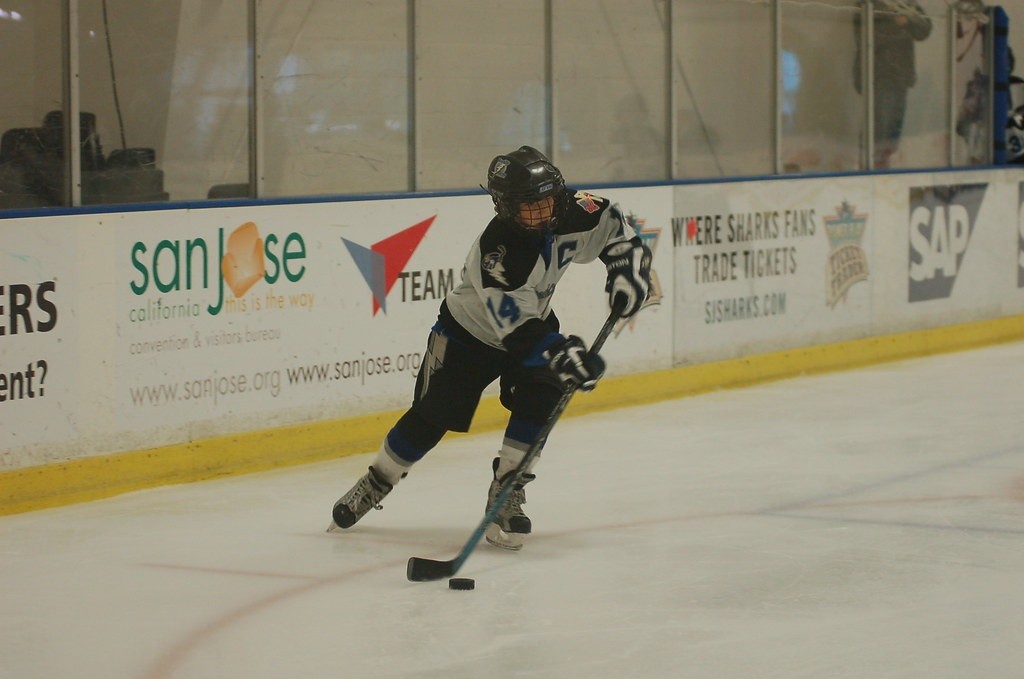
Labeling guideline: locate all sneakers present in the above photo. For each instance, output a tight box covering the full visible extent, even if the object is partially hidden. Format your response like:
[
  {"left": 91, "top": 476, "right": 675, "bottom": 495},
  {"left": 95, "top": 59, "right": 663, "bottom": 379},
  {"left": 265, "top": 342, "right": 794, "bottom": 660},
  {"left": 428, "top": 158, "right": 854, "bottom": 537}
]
[
  {"left": 485, "top": 457, "right": 536, "bottom": 551},
  {"left": 326, "top": 466, "right": 408, "bottom": 532}
]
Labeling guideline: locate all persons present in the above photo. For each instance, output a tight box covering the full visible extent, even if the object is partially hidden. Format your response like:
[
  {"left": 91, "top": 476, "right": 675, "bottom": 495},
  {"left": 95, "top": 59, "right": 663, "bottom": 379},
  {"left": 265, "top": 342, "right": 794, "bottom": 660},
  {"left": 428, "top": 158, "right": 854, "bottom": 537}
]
[
  {"left": 16, "top": 110, "right": 87, "bottom": 204},
  {"left": 331, "top": 145, "right": 651, "bottom": 536},
  {"left": 851, "top": 0, "right": 932, "bottom": 172},
  {"left": 955, "top": 1, "right": 1014, "bottom": 168}
]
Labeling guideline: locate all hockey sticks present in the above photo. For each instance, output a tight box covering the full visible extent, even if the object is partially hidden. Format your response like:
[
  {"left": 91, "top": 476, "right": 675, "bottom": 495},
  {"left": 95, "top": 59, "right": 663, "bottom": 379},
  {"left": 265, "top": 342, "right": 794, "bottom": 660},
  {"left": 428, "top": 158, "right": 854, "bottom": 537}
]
[{"left": 404, "top": 292, "right": 629, "bottom": 585}]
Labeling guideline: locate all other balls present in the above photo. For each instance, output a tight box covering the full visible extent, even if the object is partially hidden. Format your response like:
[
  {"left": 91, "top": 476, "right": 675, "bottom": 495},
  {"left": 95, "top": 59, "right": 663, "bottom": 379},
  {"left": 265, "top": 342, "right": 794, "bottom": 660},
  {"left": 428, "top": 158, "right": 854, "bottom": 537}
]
[{"left": 447, "top": 578, "right": 476, "bottom": 591}]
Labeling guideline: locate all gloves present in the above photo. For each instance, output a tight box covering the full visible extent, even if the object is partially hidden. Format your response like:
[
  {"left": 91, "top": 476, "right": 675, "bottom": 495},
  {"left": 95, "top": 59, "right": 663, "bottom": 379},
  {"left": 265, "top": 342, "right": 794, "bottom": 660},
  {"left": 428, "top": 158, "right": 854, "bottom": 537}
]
[
  {"left": 599, "top": 236, "right": 653, "bottom": 319},
  {"left": 542, "top": 335, "right": 606, "bottom": 393}
]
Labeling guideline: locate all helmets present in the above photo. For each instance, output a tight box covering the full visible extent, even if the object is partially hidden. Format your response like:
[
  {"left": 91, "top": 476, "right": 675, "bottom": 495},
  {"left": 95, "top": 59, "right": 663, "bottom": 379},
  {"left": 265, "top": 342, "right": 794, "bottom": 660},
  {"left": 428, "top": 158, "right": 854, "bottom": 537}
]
[{"left": 486, "top": 145, "right": 569, "bottom": 235}]
